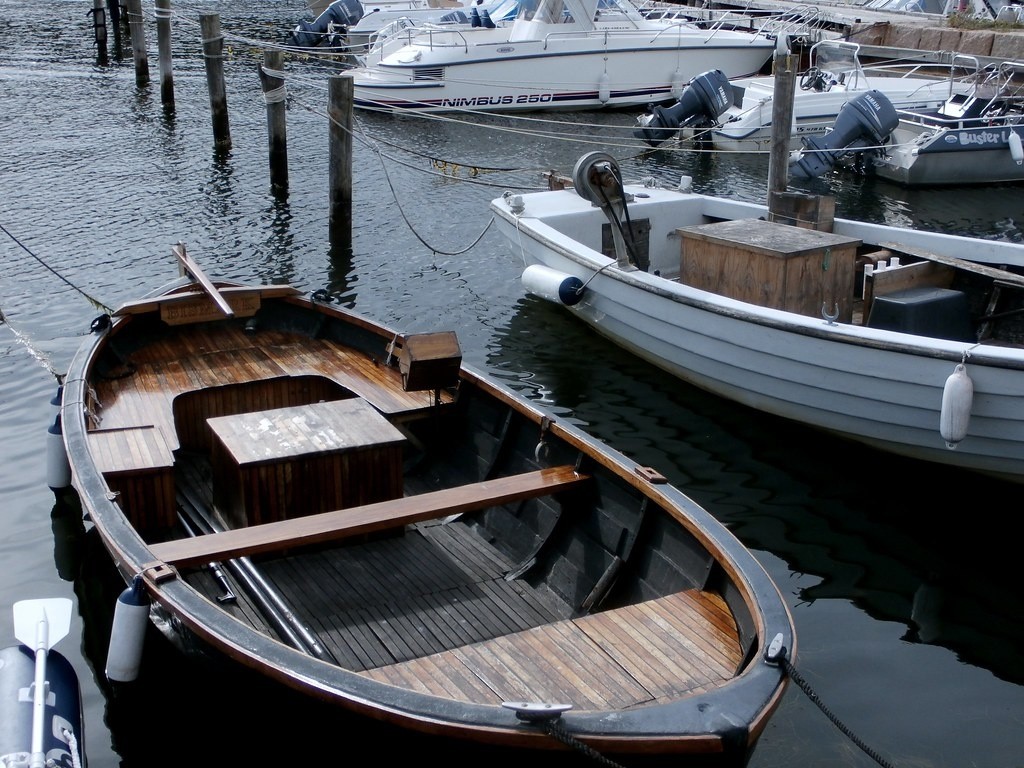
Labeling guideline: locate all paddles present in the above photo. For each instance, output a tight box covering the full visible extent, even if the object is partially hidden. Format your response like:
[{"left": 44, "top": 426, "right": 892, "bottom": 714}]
[{"left": 170, "top": 454, "right": 338, "bottom": 664}]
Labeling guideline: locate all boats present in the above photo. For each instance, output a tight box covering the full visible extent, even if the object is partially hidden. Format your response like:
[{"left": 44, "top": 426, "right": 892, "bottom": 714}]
[
  {"left": 289, "top": 0, "right": 785, "bottom": 118},
  {"left": 786, "top": 62, "right": 1024, "bottom": 186},
  {"left": 631, "top": 39, "right": 1003, "bottom": 153},
  {"left": 491, "top": 146, "right": 1024, "bottom": 476},
  {"left": 59, "top": 281, "right": 795, "bottom": 768}
]
